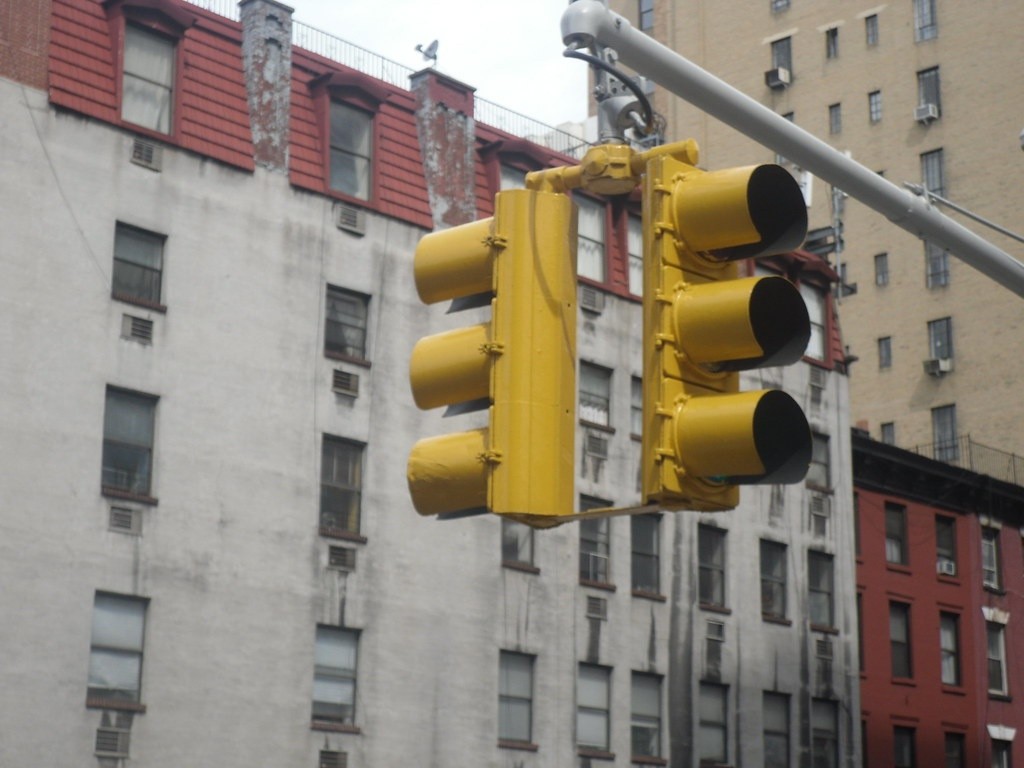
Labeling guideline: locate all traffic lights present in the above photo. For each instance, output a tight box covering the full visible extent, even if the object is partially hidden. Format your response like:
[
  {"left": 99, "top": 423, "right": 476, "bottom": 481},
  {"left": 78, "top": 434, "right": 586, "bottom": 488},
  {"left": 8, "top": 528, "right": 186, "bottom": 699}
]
[
  {"left": 406, "top": 192, "right": 582, "bottom": 531},
  {"left": 640, "top": 154, "right": 818, "bottom": 513}
]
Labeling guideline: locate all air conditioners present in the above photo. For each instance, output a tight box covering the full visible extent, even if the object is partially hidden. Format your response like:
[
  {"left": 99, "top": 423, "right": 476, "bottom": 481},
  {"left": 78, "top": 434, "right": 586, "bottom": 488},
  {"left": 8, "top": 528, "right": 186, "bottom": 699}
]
[
  {"left": 914, "top": 104, "right": 938, "bottom": 122},
  {"left": 924, "top": 359, "right": 949, "bottom": 375},
  {"left": 937, "top": 560, "right": 955, "bottom": 575},
  {"left": 764, "top": 67, "right": 790, "bottom": 87}
]
